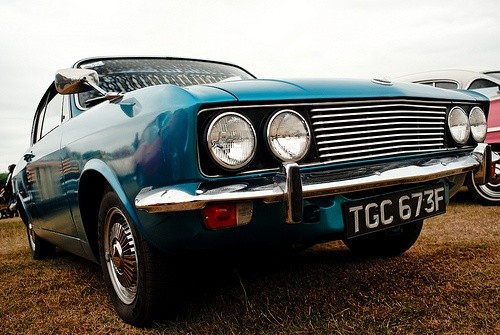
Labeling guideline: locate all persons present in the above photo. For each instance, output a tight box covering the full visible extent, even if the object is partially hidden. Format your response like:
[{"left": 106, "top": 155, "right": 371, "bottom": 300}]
[{"left": 1, "top": 164, "right": 20, "bottom": 219}]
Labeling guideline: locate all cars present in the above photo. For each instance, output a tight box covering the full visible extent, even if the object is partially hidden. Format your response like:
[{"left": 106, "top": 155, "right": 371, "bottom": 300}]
[
  {"left": 11, "top": 57, "right": 490, "bottom": 326},
  {"left": 391, "top": 68, "right": 500, "bottom": 205}
]
